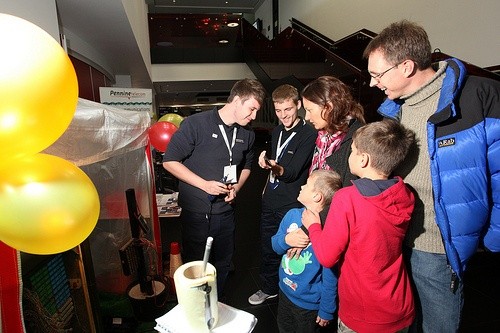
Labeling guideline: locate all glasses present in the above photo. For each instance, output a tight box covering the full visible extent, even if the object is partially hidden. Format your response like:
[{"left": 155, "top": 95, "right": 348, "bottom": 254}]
[{"left": 370, "top": 59, "right": 417, "bottom": 79}]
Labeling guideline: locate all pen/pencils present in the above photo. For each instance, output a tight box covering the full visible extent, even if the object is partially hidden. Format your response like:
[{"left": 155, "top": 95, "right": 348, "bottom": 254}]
[{"left": 199, "top": 236, "right": 213, "bottom": 276}]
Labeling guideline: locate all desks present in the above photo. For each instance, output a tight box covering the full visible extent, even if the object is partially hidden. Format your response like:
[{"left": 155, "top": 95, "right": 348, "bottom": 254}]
[{"left": 99, "top": 192, "right": 182, "bottom": 232}]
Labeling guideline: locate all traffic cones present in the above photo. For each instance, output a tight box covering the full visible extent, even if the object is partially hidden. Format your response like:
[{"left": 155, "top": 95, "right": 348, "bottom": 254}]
[{"left": 169, "top": 242, "right": 184, "bottom": 296}]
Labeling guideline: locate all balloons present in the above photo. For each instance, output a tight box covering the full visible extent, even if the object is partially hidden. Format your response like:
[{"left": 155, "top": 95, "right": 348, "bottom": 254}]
[
  {"left": 0, "top": 13, "right": 78, "bottom": 153},
  {"left": 0, "top": 153, "right": 101, "bottom": 255},
  {"left": 148, "top": 121, "right": 178, "bottom": 152},
  {"left": 158, "top": 114, "right": 183, "bottom": 128}
]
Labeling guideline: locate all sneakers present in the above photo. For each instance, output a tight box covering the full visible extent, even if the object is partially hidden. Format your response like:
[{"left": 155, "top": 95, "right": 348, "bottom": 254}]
[{"left": 248, "top": 290, "right": 279, "bottom": 305}]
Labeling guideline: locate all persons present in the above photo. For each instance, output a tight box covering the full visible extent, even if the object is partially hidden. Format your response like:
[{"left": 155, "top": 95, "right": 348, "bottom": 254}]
[
  {"left": 249, "top": 85, "right": 319, "bottom": 305},
  {"left": 301, "top": 120, "right": 421, "bottom": 333},
  {"left": 163, "top": 79, "right": 266, "bottom": 302},
  {"left": 301, "top": 76, "right": 366, "bottom": 188},
  {"left": 271, "top": 169, "right": 342, "bottom": 333},
  {"left": 363, "top": 20, "right": 500, "bottom": 333}
]
